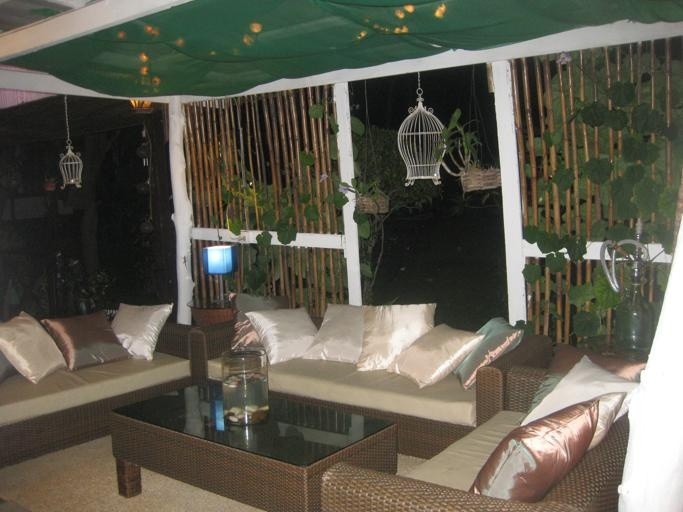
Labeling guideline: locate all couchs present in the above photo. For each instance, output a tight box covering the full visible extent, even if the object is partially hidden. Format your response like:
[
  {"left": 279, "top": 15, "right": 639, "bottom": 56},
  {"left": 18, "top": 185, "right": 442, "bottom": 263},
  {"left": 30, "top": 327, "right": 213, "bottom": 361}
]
[
  {"left": 189, "top": 317, "right": 553, "bottom": 460},
  {"left": 1, "top": 322, "right": 207, "bottom": 469},
  {"left": 321, "top": 365, "right": 630, "bottom": 512}
]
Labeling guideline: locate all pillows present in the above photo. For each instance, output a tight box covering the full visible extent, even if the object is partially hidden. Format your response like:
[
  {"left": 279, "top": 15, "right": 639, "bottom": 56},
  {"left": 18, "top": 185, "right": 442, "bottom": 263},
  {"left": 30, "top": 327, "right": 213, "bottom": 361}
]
[
  {"left": 0, "top": 310, "right": 67, "bottom": 384},
  {"left": 529, "top": 373, "right": 564, "bottom": 419},
  {"left": 387, "top": 323, "right": 485, "bottom": 390},
  {"left": 468, "top": 399, "right": 599, "bottom": 503},
  {"left": 244, "top": 308, "right": 319, "bottom": 365},
  {"left": 41, "top": 311, "right": 132, "bottom": 372},
  {"left": 110, "top": 302, "right": 174, "bottom": 361},
  {"left": 586, "top": 392, "right": 627, "bottom": 451},
  {"left": 357, "top": 303, "right": 437, "bottom": 372},
  {"left": 303, "top": 303, "right": 363, "bottom": 363},
  {"left": 228, "top": 292, "right": 289, "bottom": 356},
  {"left": 520, "top": 354, "right": 640, "bottom": 427},
  {"left": 453, "top": 317, "right": 525, "bottom": 391}
]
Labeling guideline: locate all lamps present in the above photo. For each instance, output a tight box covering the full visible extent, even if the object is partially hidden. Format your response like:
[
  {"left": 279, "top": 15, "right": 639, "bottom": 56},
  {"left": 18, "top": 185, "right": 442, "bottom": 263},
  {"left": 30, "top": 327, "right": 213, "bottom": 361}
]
[{"left": 202, "top": 243, "right": 237, "bottom": 304}]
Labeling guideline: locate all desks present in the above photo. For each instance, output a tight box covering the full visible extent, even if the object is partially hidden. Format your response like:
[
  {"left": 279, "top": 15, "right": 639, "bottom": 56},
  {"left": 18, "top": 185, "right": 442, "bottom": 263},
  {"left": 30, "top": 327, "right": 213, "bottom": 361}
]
[{"left": 187, "top": 296, "right": 236, "bottom": 360}]
